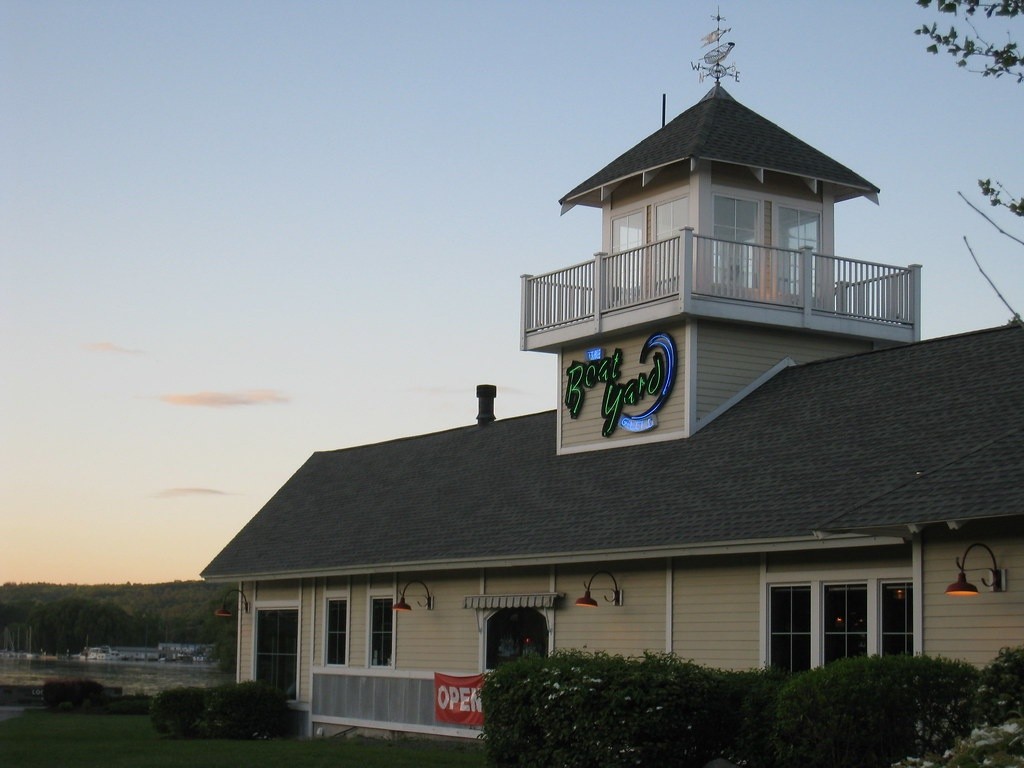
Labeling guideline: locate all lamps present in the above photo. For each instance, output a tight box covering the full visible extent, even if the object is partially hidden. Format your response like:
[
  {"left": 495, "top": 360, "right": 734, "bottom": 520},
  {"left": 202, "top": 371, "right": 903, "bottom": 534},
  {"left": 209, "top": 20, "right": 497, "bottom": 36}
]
[
  {"left": 575, "top": 571, "right": 623, "bottom": 608},
  {"left": 391, "top": 580, "right": 434, "bottom": 612},
  {"left": 214, "top": 590, "right": 250, "bottom": 616},
  {"left": 945, "top": 543, "right": 1008, "bottom": 596}
]
